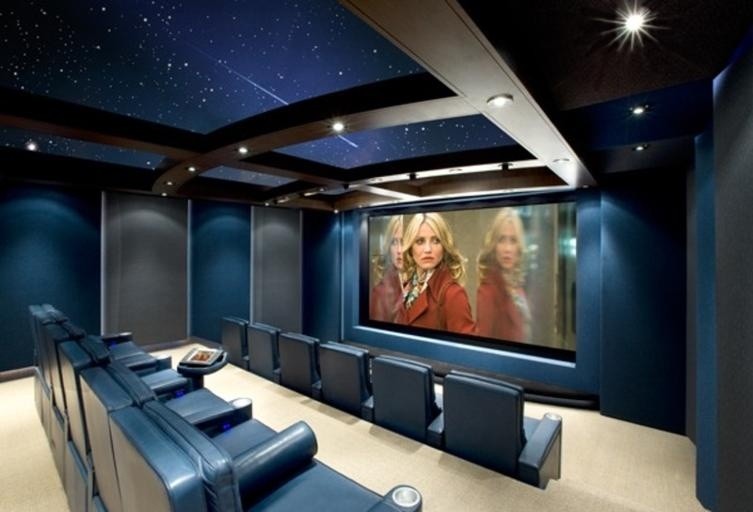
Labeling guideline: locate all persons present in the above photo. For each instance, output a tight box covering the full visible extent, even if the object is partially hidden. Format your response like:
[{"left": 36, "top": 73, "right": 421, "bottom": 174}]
[
  {"left": 369, "top": 214, "right": 407, "bottom": 324},
  {"left": 475, "top": 207, "right": 538, "bottom": 344},
  {"left": 390, "top": 212, "right": 477, "bottom": 337}
]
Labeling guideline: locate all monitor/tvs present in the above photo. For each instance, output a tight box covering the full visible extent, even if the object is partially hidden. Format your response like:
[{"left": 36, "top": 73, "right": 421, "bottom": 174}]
[{"left": 357, "top": 200, "right": 578, "bottom": 363}]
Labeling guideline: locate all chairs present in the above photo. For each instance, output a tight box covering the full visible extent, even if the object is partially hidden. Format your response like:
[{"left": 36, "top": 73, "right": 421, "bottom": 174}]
[
  {"left": 218, "top": 314, "right": 559, "bottom": 492},
  {"left": 29, "top": 303, "right": 424, "bottom": 508}
]
